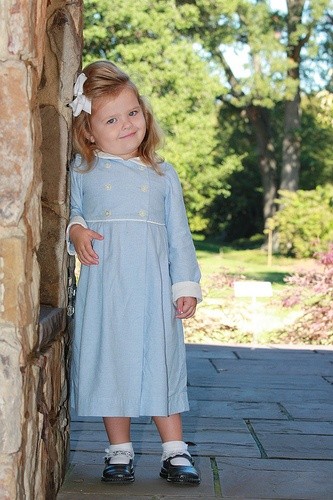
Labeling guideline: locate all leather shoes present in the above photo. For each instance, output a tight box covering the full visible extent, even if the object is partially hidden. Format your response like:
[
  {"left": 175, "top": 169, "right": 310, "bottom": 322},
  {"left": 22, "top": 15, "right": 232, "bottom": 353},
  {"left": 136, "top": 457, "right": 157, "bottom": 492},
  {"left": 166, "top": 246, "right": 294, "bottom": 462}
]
[
  {"left": 101, "top": 452, "right": 135, "bottom": 483},
  {"left": 158, "top": 454, "right": 200, "bottom": 485}
]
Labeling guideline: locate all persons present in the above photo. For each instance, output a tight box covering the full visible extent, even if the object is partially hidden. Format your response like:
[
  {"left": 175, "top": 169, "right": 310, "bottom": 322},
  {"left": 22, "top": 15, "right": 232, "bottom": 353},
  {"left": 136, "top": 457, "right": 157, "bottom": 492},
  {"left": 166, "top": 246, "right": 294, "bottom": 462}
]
[{"left": 62, "top": 60, "right": 205, "bottom": 490}]
